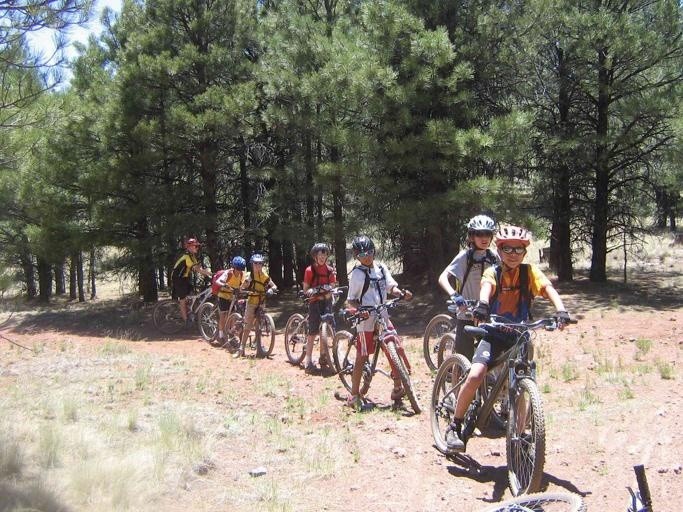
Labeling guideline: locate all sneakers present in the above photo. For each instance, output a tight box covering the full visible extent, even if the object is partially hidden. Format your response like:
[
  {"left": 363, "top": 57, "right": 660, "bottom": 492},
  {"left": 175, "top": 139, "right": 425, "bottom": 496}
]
[{"left": 218, "top": 336, "right": 466, "bottom": 454}]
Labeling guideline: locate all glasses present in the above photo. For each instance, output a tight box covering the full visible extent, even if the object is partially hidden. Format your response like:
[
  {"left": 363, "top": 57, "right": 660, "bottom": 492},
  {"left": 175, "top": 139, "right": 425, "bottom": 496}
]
[
  {"left": 476, "top": 231, "right": 492, "bottom": 237},
  {"left": 502, "top": 245, "right": 525, "bottom": 253}
]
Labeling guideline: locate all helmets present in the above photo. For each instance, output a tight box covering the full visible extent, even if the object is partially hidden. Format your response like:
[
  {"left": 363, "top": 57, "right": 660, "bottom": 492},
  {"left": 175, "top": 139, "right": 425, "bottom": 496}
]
[
  {"left": 233, "top": 236, "right": 374, "bottom": 271},
  {"left": 183, "top": 238, "right": 201, "bottom": 247},
  {"left": 495, "top": 227, "right": 530, "bottom": 246},
  {"left": 468, "top": 215, "right": 496, "bottom": 231}
]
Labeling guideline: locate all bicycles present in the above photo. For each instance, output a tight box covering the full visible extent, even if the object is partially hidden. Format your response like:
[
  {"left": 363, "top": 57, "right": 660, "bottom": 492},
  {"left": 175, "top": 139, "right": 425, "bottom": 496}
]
[
  {"left": 152, "top": 272, "right": 213, "bottom": 334},
  {"left": 196, "top": 283, "right": 244, "bottom": 355},
  {"left": 283, "top": 286, "right": 349, "bottom": 372},
  {"left": 484, "top": 462, "right": 657, "bottom": 512},
  {"left": 429, "top": 313, "right": 577, "bottom": 492},
  {"left": 330, "top": 289, "right": 422, "bottom": 413},
  {"left": 436, "top": 299, "right": 535, "bottom": 436},
  {"left": 421, "top": 299, "right": 457, "bottom": 383},
  {"left": 223, "top": 292, "right": 275, "bottom": 358}
]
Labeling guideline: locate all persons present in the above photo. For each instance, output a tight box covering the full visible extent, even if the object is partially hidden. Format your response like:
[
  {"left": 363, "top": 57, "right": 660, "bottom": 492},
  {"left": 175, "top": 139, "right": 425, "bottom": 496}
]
[
  {"left": 301, "top": 241, "right": 336, "bottom": 375},
  {"left": 211, "top": 254, "right": 248, "bottom": 345},
  {"left": 344, "top": 235, "right": 412, "bottom": 410},
  {"left": 444, "top": 226, "right": 570, "bottom": 452},
  {"left": 168, "top": 238, "right": 210, "bottom": 328},
  {"left": 436, "top": 214, "right": 499, "bottom": 375},
  {"left": 233, "top": 253, "right": 278, "bottom": 359}
]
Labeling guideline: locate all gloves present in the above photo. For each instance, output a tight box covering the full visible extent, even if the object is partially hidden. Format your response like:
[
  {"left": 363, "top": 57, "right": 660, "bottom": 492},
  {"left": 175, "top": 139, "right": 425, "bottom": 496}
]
[
  {"left": 451, "top": 292, "right": 464, "bottom": 305},
  {"left": 556, "top": 311, "right": 571, "bottom": 327},
  {"left": 473, "top": 301, "right": 490, "bottom": 321}
]
